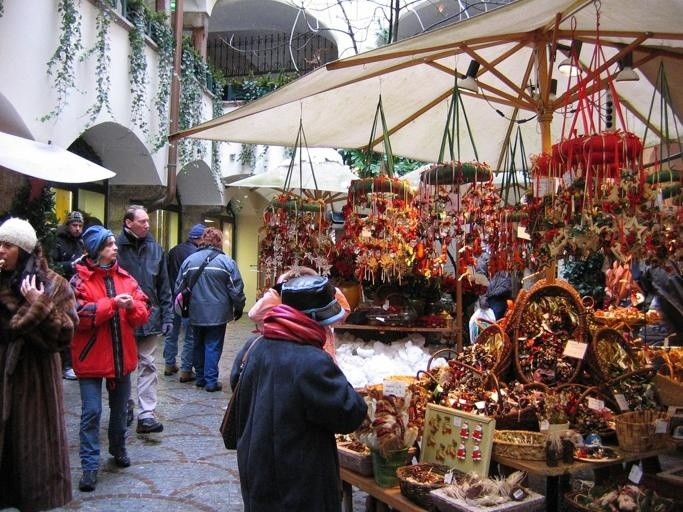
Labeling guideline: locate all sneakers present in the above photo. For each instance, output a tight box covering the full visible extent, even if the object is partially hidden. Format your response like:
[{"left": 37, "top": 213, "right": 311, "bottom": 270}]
[
  {"left": 64, "top": 370, "right": 77, "bottom": 379},
  {"left": 109, "top": 447, "right": 130, "bottom": 467},
  {"left": 79, "top": 470, "right": 96, "bottom": 491},
  {"left": 127, "top": 400, "right": 134, "bottom": 424},
  {"left": 163, "top": 360, "right": 226, "bottom": 393},
  {"left": 136, "top": 418, "right": 164, "bottom": 434}
]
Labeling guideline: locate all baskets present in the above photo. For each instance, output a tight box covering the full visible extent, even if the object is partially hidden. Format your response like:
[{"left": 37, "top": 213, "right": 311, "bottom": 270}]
[
  {"left": 411, "top": 274, "right": 683, "bottom": 461},
  {"left": 563, "top": 479, "right": 672, "bottom": 512},
  {"left": 355, "top": 273, "right": 683, "bottom": 512},
  {"left": 428, "top": 480, "right": 547, "bottom": 512},
  {"left": 358, "top": 389, "right": 403, "bottom": 450},
  {"left": 396, "top": 462, "right": 483, "bottom": 512}
]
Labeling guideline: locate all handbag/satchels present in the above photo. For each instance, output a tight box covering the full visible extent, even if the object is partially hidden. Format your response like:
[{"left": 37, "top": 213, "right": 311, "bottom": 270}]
[
  {"left": 174, "top": 287, "right": 192, "bottom": 318},
  {"left": 218, "top": 373, "right": 241, "bottom": 449}
]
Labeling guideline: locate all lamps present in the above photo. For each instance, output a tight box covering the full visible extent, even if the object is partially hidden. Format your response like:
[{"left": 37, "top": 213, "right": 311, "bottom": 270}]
[
  {"left": 558, "top": 39, "right": 583, "bottom": 76},
  {"left": 456, "top": 59, "right": 480, "bottom": 95},
  {"left": 548, "top": 79, "right": 558, "bottom": 100},
  {"left": 615, "top": 46, "right": 639, "bottom": 82}
]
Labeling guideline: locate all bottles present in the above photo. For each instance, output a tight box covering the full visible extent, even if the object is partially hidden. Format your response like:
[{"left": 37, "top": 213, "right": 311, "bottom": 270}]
[{"left": 573, "top": 445, "right": 624, "bottom": 463}]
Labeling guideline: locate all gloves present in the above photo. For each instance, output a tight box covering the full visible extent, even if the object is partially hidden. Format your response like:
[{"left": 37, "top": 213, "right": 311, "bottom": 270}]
[
  {"left": 234, "top": 308, "right": 242, "bottom": 321},
  {"left": 162, "top": 324, "right": 174, "bottom": 336}
]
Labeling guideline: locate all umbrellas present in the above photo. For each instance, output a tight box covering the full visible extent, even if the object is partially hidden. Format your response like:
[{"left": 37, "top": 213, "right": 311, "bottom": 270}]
[{"left": 164, "top": 1, "right": 683, "bottom": 193}]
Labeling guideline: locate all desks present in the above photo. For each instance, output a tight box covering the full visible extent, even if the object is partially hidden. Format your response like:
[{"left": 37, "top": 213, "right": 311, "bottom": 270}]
[
  {"left": 339, "top": 461, "right": 547, "bottom": 512},
  {"left": 498, "top": 451, "right": 664, "bottom": 493}
]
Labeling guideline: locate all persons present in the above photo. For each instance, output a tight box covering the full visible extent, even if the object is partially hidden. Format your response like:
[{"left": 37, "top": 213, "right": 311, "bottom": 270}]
[
  {"left": 163, "top": 223, "right": 246, "bottom": 392},
  {"left": 230, "top": 267, "right": 318, "bottom": 392},
  {"left": 1, "top": 204, "right": 172, "bottom": 511},
  {"left": 230, "top": 275, "right": 368, "bottom": 512}
]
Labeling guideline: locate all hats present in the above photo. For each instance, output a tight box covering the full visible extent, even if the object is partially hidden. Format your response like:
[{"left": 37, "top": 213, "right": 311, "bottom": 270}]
[
  {"left": 281, "top": 276, "right": 345, "bottom": 325},
  {"left": 67, "top": 211, "right": 84, "bottom": 223},
  {"left": 0, "top": 218, "right": 37, "bottom": 255},
  {"left": 81, "top": 226, "right": 114, "bottom": 258},
  {"left": 188, "top": 223, "right": 206, "bottom": 239}
]
